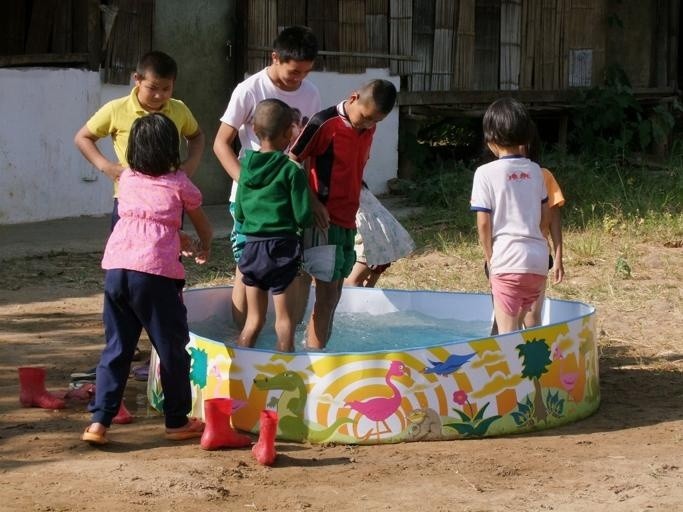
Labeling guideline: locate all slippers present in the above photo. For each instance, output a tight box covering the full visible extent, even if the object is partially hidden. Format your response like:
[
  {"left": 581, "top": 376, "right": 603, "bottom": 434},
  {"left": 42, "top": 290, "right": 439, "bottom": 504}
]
[
  {"left": 132, "top": 359, "right": 150, "bottom": 380},
  {"left": 160, "top": 416, "right": 206, "bottom": 440},
  {"left": 69, "top": 369, "right": 97, "bottom": 390},
  {"left": 79, "top": 425, "right": 109, "bottom": 445}
]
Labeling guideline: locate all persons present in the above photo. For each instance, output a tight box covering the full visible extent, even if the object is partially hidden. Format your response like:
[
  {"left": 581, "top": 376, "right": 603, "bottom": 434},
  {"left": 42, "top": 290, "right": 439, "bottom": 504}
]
[
  {"left": 485, "top": 142, "right": 567, "bottom": 338},
  {"left": 345, "top": 181, "right": 417, "bottom": 286},
  {"left": 233, "top": 97, "right": 314, "bottom": 353},
  {"left": 468, "top": 97, "right": 551, "bottom": 334},
  {"left": 287, "top": 77, "right": 396, "bottom": 350},
  {"left": 79, "top": 111, "right": 214, "bottom": 447},
  {"left": 73, "top": 50, "right": 204, "bottom": 380},
  {"left": 212, "top": 24, "right": 321, "bottom": 332}
]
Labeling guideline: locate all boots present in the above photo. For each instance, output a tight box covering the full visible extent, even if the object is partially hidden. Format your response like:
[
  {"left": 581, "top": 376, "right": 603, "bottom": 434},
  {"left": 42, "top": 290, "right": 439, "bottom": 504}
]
[
  {"left": 199, "top": 397, "right": 252, "bottom": 451},
  {"left": 111, "top": 398, "right": 133, "bottom": 423},
  {"left": 251, "top": 409, "right": 279, "bottom": 466},
  {"left": 17, "top": 364, "right": 68, "bottom": 409}
]
